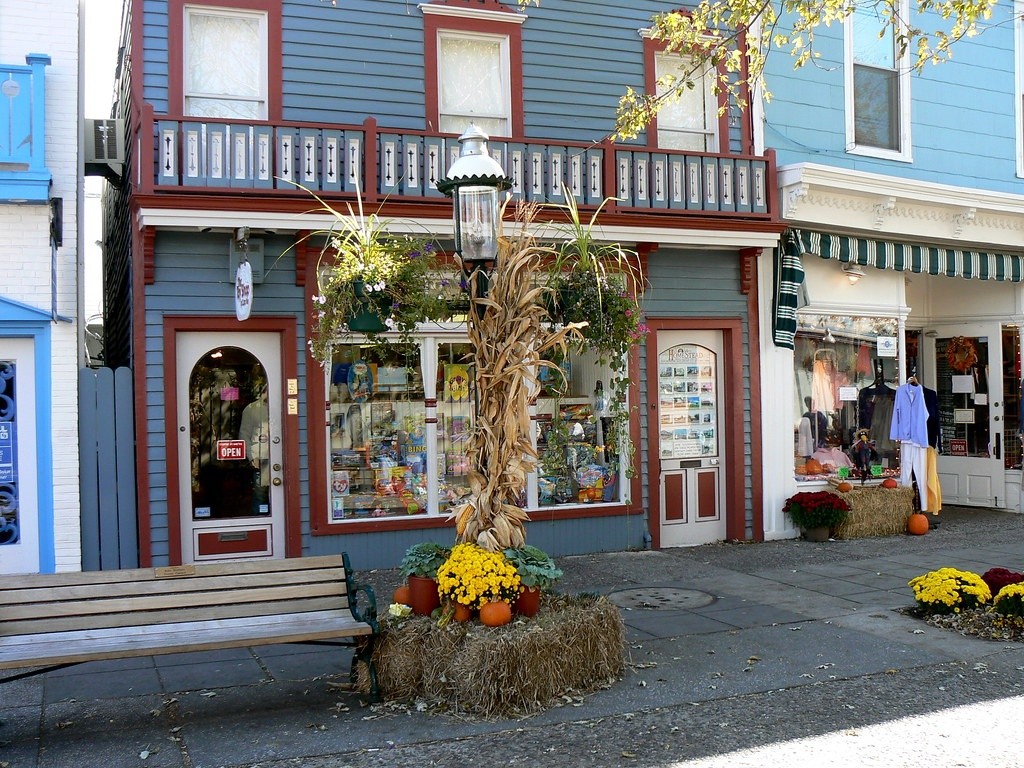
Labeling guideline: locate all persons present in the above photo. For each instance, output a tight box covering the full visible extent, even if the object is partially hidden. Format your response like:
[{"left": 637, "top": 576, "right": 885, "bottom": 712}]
[
  {"left": 801, "top": 396, "right": 827, "bottom": 448},
  {"left": 855, "top": 428, "right": 878, "bottom": 476},
  {"left": 237, "top": 385, "right": 271, "bottom": 515}
]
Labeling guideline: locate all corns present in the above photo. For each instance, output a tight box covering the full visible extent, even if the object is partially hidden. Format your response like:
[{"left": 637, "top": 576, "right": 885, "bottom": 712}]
[{"left": 455, "top": 505, "right": 476, "bottom": 536}]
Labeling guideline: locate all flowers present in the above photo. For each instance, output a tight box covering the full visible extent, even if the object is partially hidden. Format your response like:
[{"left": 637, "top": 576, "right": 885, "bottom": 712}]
[
  {"left": 436, "top": 541, "right": 523, "bottom": 610},
  {"left": 781, "top": 491, "right": 852, "bottom": 529},
  {"left": 529, "top": 182, "right": 652, "bottom": 553},
  {"left": 265, "top": 168, "right": 470, "bottom": 438},
  {"left": 945, "top": 335, "right": 976, "bottom": 370}
]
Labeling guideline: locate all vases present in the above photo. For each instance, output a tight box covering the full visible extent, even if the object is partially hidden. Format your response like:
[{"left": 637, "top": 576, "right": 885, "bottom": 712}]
[
  {"left": 561, "top": 307, "right": 602, "bottom": 339},
  {"left": 338, "top": 281, "right": 394, "bottom": 332},
  {"left": 805, "top": 527, "right": 829, "bottom": 542}
]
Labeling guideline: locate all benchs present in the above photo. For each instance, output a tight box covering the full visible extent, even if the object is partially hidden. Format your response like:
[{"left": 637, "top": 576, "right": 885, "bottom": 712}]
[{"left": 1, "top": 551, "right": 381, "bottom": 701}]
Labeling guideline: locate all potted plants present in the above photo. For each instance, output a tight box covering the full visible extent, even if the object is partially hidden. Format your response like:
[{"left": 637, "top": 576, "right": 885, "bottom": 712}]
[
  {"left": 503, "top": 545, "right": 565, "bottom": 618},
  {"left": 397, "top": 543, "right": 451, "bottom": 615}
]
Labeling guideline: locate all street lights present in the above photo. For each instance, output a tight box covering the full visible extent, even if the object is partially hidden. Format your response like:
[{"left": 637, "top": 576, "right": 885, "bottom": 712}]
[{"left": 433, "top": 121, "right": 514, "bottom": 323}]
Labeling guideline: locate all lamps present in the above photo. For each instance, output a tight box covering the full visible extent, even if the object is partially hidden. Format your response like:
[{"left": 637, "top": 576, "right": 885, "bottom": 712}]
[
  {"left": 840, "top": 262, "right": 866, "bottom": 286},
  {"left": 823, "top": 328, "right": 836, "bottom": 343}
]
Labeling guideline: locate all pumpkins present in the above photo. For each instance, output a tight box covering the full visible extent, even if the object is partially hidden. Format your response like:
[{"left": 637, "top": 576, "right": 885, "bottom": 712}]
[
  {"left": 908, "top": 511, "right": 929, "bottom": 535},
  {"left": 393, "top": 579, "right": 409, "bottom": 604},
  {"left": 806, "top": 458, "right": 822, "bottom": 474},
  {"left": 480, "top": 594, "right": 511, "bottom": 626},
  {"left": 883, "top": 477, "right": 897, "bottom": 488},
  {"left": 431, "top": 595, "right": 472, "bottom": 629},
  {"left": 837, "top": 480, "right": 851, "bottom": 492}
]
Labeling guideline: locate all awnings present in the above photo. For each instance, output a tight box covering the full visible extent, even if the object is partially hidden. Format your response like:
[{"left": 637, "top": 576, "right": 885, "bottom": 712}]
[{"left": 771, "top": 226, "right": 1024, "bottom": 351}]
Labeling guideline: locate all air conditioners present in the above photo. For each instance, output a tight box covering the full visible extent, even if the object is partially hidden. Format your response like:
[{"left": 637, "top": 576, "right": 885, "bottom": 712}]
[{"left": 84, "top": 119, "right": 125, "bottom": 175}]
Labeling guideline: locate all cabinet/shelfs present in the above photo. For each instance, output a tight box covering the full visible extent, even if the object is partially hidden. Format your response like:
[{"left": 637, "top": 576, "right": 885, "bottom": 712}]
[{"left": 1003, "top": 377, "right": 1018, "bottom": 417}]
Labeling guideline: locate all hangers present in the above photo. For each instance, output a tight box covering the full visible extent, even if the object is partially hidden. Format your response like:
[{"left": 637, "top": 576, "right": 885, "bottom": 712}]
[
  {"left": 906, "top": 373, "right": 920, "bottom": 385},
  {"left": 867, "top": 372, "right": 890, "bottom": 389}
]
